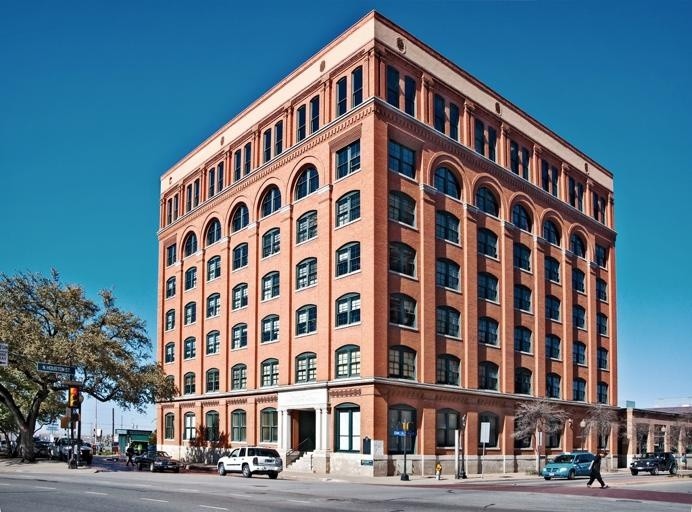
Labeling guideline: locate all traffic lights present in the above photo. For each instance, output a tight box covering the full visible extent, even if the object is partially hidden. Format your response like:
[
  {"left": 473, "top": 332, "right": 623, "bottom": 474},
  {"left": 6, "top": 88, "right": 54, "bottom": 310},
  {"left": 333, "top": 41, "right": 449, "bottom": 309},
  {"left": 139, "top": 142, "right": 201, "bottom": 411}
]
[{"left": 70, "top": 387, "right": 79, "bottom": 407}]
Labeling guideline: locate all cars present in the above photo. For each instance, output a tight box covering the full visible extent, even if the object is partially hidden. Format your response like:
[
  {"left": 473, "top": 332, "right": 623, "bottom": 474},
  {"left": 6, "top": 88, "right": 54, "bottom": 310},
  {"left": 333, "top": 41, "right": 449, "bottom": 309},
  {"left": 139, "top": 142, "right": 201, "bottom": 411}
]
[
  {"left": 135, "top": 451, "right": 179, "bottom": 472},
  {"left": 217, "top": 447, "right": 283, "bottom": 478},
  {"left": 630, "top": 452, "right": 678, "bottom": 475},
  {"left": 542, "top": 453, "right": 596, "bottom": 479},
  {"left": 50, "top": 438, "right": 93, "bottom": 464}
]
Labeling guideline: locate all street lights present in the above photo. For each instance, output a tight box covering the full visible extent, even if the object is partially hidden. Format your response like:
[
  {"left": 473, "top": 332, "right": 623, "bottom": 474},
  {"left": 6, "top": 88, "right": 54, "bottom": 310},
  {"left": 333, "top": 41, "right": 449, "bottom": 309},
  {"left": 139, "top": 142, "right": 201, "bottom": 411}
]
[
  {"left": 459, "top": 414, "right": 466, "bottom": 478},
  {"left": 401, "top": 416, "right": 410, "bottom": 480}
]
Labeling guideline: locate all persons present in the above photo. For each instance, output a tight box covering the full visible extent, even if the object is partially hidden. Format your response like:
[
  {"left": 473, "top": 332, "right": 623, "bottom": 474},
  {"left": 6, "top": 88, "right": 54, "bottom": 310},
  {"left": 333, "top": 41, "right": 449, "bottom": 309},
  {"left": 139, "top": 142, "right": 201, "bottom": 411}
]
[
  {"left": 125, "top": 445, "right": 135, "bottom": 466},
  {"left": 587, "top": 452, "right": 608, "bottom": 489}
]
